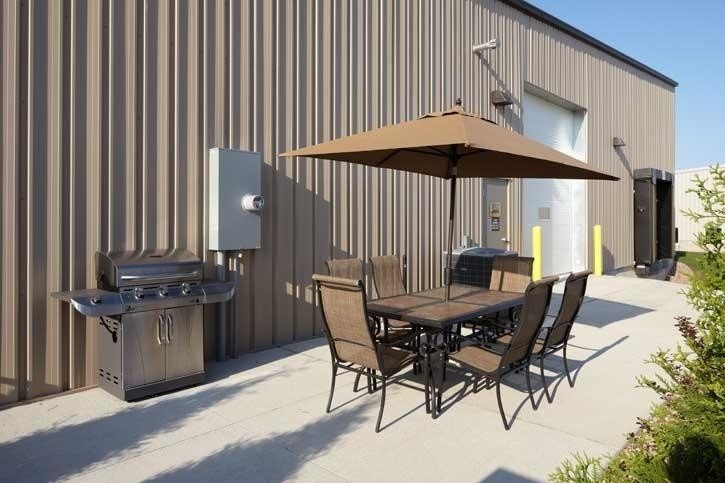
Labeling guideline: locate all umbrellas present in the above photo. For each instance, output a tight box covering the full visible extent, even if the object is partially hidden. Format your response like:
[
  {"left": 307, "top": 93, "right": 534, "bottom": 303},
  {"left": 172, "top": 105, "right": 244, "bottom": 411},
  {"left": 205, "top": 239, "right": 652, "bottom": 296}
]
[{"left": 271, "top": 96, "right": 621, "bottom": 296}]
